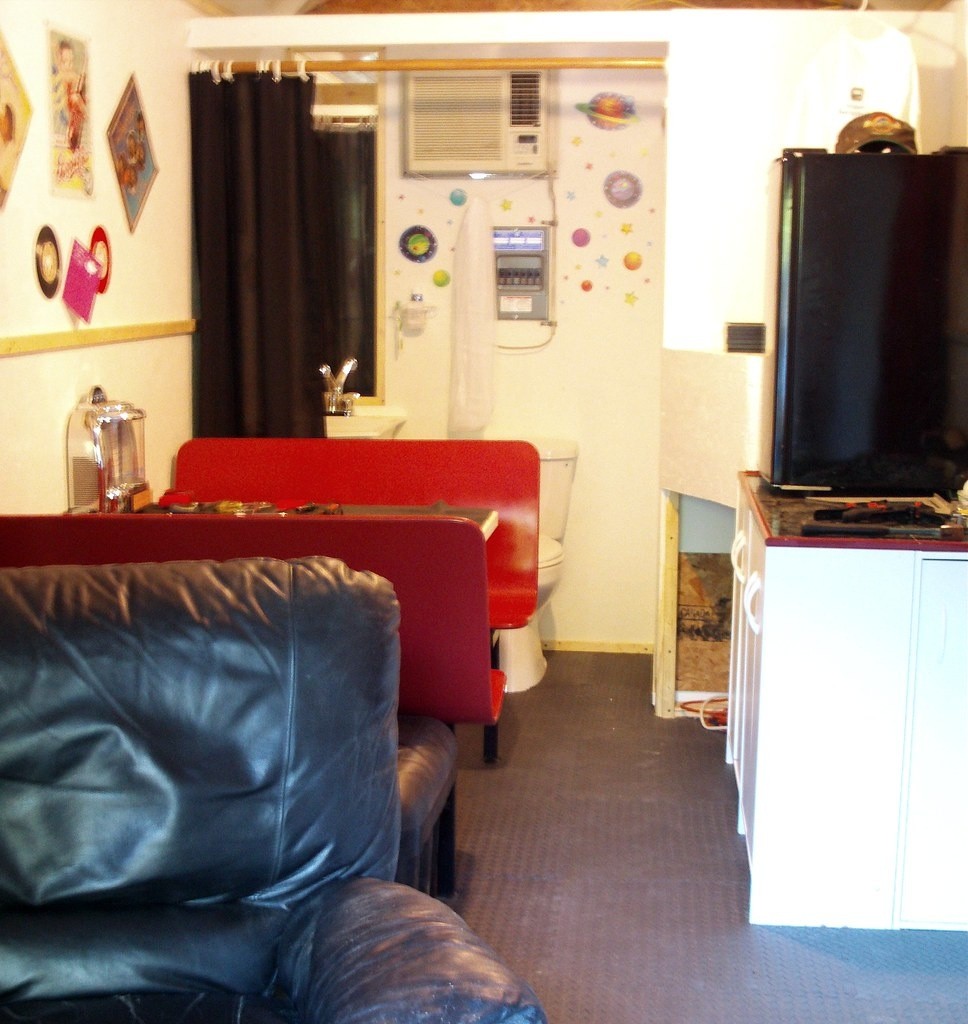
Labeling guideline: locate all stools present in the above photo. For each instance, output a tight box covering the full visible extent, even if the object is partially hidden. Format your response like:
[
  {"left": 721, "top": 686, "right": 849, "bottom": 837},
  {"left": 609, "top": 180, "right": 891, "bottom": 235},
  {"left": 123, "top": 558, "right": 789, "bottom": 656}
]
[{"left": 397, "top": 713, "right": 459, "bottom": 897}]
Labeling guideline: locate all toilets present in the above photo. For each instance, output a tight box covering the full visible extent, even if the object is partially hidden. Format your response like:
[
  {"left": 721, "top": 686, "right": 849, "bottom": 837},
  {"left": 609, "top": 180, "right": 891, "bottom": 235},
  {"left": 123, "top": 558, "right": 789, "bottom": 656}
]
[{"left": 453, "top": 436, "right": 578, "bottom": 695}]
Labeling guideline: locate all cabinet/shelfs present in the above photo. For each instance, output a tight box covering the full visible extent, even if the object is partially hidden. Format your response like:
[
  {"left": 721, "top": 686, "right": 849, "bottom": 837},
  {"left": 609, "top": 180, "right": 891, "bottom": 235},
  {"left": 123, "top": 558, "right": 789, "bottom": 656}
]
[{"left": 725, "top": 486, "right": 767, "bottom": 872}]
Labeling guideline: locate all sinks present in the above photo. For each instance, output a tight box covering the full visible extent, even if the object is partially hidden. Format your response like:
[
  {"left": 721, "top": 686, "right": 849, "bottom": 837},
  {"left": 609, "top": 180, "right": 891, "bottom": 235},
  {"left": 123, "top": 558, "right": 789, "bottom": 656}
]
[{"left": 323, "top": 416, "right": 410, "bottom": 439}]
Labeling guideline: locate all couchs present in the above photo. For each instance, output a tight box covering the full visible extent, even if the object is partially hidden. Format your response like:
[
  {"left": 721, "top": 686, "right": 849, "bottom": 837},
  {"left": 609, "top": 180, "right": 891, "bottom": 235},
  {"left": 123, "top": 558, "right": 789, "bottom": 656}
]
[{"left": 0, "top": 554, "right": 549, "bottom": 1024}]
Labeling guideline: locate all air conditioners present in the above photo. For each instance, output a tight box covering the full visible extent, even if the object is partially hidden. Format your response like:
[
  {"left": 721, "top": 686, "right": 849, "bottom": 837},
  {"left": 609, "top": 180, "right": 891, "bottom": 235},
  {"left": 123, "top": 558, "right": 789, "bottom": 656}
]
[{"left": 403, "top": 70, "right": 549, "bottom": 178}]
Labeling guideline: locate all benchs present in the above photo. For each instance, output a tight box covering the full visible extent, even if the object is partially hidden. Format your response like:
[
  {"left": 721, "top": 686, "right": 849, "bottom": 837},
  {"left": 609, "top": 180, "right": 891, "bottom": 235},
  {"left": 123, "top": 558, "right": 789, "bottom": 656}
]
[
  {"left": 173, "top": 438, "right": 541, "bottom": 646},
  {"left": 0, "top": 514, "right": 506, "bottom": 898}
]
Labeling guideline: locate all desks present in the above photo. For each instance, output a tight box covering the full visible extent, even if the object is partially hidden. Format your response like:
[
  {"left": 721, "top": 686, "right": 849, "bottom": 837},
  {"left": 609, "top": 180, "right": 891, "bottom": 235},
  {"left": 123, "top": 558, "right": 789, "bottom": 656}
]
[{"left": 136, "top": 502, "right": 499, "bottom": 542}]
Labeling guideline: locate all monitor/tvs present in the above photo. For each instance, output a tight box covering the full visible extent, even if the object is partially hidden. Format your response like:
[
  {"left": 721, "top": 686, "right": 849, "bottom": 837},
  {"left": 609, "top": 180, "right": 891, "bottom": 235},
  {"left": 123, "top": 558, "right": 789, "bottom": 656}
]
[{"left": 758, "top": 145, "right": 968, "bottom": 498}]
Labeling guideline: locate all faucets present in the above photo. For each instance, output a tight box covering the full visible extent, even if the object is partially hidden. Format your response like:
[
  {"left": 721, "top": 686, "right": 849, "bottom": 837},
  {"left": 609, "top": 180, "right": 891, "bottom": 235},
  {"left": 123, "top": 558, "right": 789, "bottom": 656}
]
[
  {"left": 318, "top": 363, "right": 353, "bottom": 417},
  {"left": 334, "top": 357, "right": 360, "bottom": 400}
]
